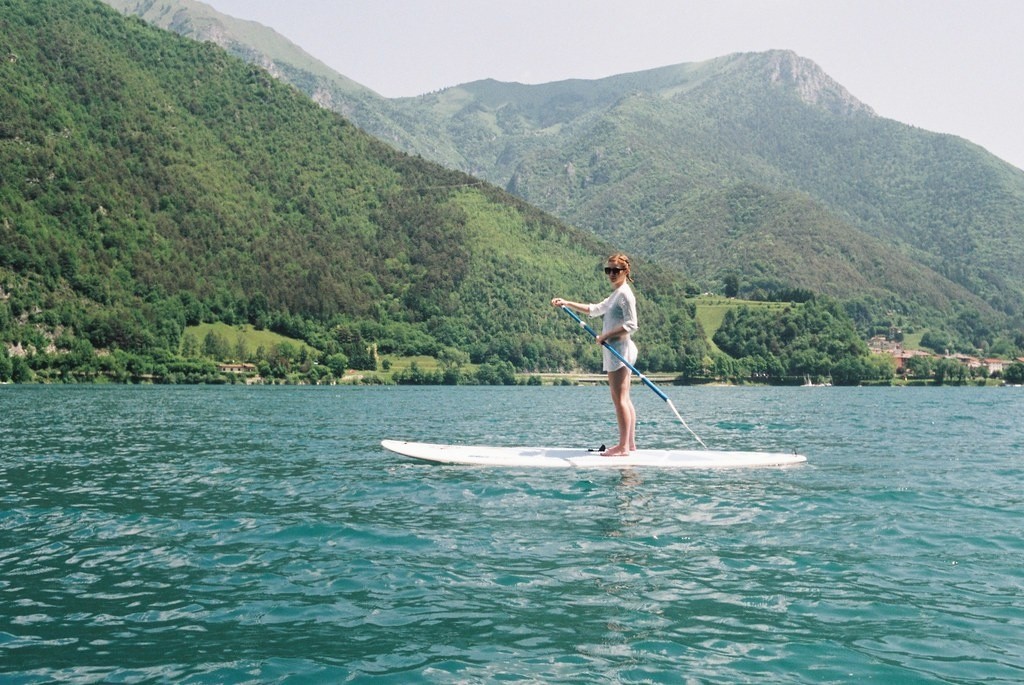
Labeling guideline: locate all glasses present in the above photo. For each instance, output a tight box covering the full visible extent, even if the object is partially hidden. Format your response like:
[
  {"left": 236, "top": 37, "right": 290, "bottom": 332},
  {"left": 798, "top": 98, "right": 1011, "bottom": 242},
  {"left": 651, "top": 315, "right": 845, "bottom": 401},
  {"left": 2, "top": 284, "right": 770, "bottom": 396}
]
[{"left": 604, "top": 267, "right": 625, "bottom": 274}]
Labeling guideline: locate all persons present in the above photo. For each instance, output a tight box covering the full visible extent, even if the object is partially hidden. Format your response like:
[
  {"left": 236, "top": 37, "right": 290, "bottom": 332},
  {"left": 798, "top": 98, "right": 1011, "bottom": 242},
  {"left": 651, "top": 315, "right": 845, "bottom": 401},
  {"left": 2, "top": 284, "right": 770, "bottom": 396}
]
[{"left": 552, "top": 253, "right": 637, "bottom": 454}]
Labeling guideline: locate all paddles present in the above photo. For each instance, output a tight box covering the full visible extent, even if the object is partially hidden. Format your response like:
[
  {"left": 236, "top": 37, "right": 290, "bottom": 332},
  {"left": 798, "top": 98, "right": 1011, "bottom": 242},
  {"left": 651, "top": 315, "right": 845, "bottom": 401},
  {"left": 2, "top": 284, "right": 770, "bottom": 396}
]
[{"left": 557, "top": 300, "right": 706, "bottom": 449}]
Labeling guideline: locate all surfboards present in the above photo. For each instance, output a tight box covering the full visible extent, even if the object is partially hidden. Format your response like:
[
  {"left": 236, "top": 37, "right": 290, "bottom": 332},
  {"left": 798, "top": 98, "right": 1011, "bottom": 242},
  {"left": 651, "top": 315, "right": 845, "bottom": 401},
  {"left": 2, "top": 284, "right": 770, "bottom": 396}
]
[{"left": 378, "top": 439, "right": 810, "bottom": 471}]
